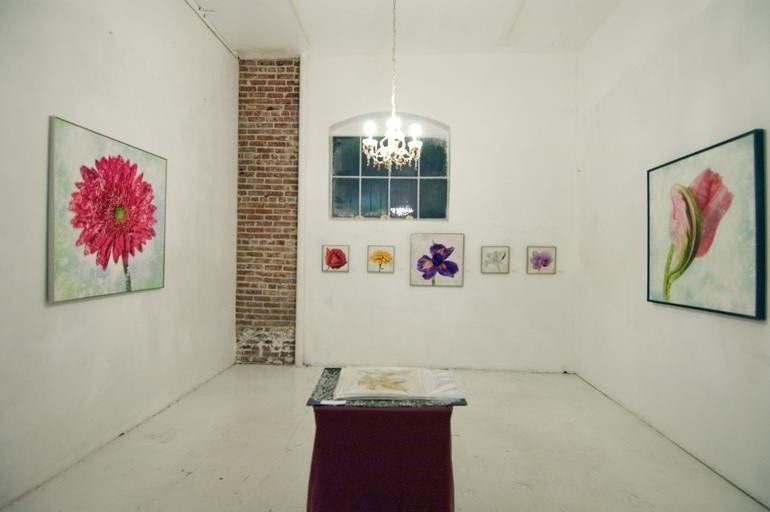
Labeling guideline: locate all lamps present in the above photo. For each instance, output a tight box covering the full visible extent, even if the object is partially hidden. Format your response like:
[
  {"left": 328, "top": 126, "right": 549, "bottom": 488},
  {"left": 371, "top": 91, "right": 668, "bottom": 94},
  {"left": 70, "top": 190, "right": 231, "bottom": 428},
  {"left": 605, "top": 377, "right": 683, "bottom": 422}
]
[{"left": 360, "top": 1, "right": 424, "bottom": 172}]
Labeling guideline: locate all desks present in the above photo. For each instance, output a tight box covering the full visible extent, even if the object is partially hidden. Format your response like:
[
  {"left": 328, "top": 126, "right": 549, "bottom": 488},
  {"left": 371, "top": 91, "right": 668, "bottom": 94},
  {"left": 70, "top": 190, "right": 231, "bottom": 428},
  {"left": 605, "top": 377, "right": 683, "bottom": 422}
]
[{"left": 308, "top": 367, "right": 466, "bottom": 511}]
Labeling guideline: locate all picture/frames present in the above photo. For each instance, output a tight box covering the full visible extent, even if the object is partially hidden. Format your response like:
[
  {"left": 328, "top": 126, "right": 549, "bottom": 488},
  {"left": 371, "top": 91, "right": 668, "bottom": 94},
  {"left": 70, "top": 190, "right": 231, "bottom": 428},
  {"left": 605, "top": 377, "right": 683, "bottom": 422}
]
[
  {"left": 321, "top": 233, "right": 557, "bottom": 286},
  {"left": 48, "top": 116, "right": 167, "bottom": 305},
  {"left": 647, "top": 129, "right": 765, "bottom": 322}
]
